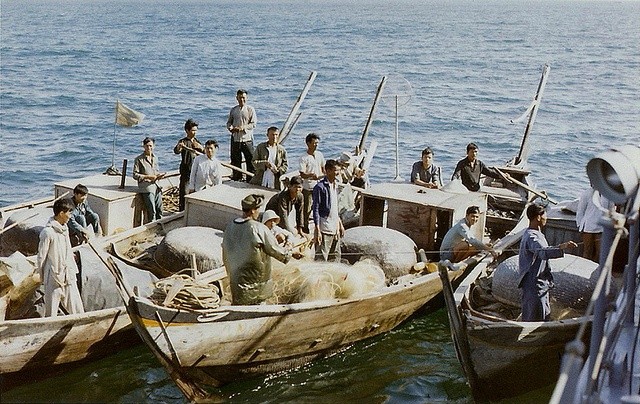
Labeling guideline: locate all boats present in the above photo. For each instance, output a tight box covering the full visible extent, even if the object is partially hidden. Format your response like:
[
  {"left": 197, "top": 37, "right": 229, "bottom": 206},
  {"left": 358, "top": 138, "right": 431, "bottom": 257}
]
[
  {"left": 0, "top": 157, "right": 248, "bottom": 274},
  {"left": 127, "top": 158, "right": 537, "bottom": 388},
  {"left": 452, "top": 198, "right": 640, "bottom": 404},
  {"left": 0, "top": 147, "right": 370, "bottom": 379}
]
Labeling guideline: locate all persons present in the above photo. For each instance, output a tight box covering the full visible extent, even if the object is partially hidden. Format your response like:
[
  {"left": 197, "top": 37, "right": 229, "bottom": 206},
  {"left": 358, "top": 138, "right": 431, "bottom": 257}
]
[
  {"left": 251, "top": 127, "right": 288, "bottom": 189},
  {"left": 576, "top": 186, "right": 615, "bottom": 262},
  {"left": 173, "top": 120, "right": 205, "bottom": 211},
  {"left": 66, "top": 183, "right": 102, "bottom": 243},
  {"left": 518, "top": 204, "right": 579, "bottom": 322},
  {"left": 259, "top": 209, "right": 293, "bottom": 246},
  {"left": 222, "top": 194, "right": 306, "bottom": 305},
  {"left": 189, "top": 140, "right": 224, "bottom": 193},
  {"left": 36, "top": 198, "right": 85, "bottom": 318},
  {"left": 264, "top": 175, "right": 305, "bottom": 238},
  {"left": 411, "top": 146, "right": 440, "bottom": 187},
  {"left": 311, "top": 158, "right": 346, "bottom": 264},
  {"left": 227, "top": 89, "right": 256, "bottom": 182},
  {"left": 438, "top": 205, "right": 501, "bottom": 259},
  {"left": 133, "top": 136, "right": 165, "bottom": 223},
  {"left": 298, "top": 133, "right": 328, "bottom": 233},
  {"left": 451, "top": 142, "right": 511, "bottom": 192}
]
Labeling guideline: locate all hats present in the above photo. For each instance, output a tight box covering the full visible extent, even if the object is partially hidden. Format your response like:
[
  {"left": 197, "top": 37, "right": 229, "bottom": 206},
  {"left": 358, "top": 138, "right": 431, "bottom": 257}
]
[{"left": 262, "top": 210, "right": 280, "bottom": 224}]
